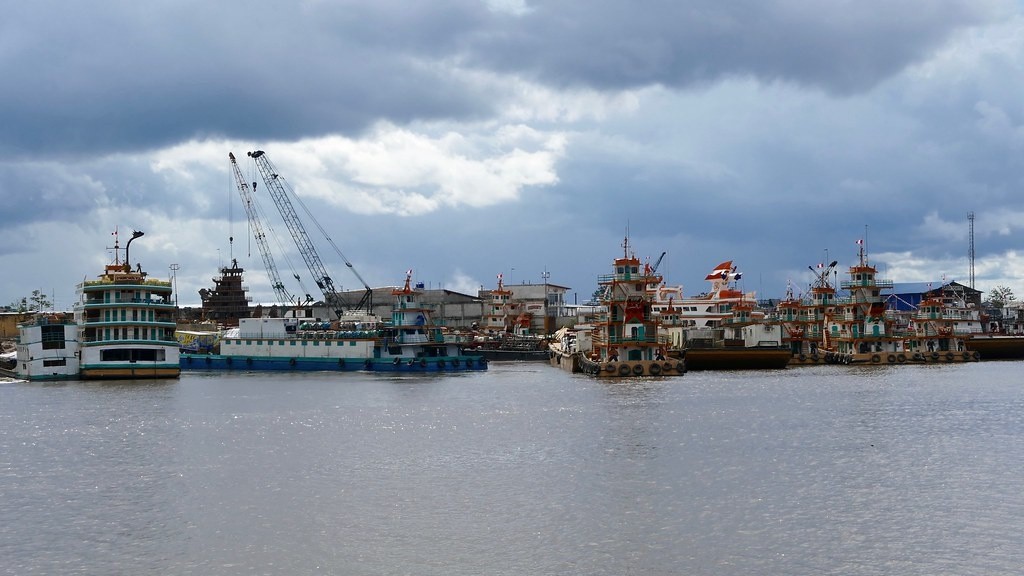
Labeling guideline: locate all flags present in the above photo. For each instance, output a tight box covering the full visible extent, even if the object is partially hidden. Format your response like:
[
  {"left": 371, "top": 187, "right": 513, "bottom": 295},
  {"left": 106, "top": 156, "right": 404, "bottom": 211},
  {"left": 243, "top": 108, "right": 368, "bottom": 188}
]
[
  {"left": 817, "top": 264, "right": 823, "bottom": 268},
  {"left": 855, "top": 239, "right": 863, "bottom": 245},
  {"left": 705, "top": 260, "right": 733, "bottom": 280}
]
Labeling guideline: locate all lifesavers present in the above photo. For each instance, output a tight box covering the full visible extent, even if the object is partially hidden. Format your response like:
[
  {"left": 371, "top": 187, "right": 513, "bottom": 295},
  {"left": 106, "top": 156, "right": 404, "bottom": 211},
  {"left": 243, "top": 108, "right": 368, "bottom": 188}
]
[
  {"left": 420, "top": 359, "right": 428, "bottom": 367},
  {"left": 619, "top": 364, "right": 631, "bottom": 375},
  {"left": 407, "top": 359, "right": 414, "bottom": 365},
  {"left": 632, "top": 364, "right": 644, "bottom": 374},
  {"left": 186, "top": 356, "right": 253, "bottom": 365},
  {"left": 798, "top": 352, "right": 981, "bottom": 365},
  {"left": 451, "top": 359, "right": 460, "bottom": 367},
  {"left": 289, "top": 359, "right": 295, "bottom": 366},
  {"left": 677, "top": 363, "right": 686, "bottom": 373},
  {"left": 604, "top": 363, "right": 616, "bottom": 372},
  {"left": 591, "top": 364, "right": 601, "bottom": 373},
  {"left": 465, "top": 358, "right": 473, "bottom": 367},
  {"left": 338, "top": 359, "right": 345, "bottom": 367},
  {"left": 394, "top": 357, "right": 401, "bottom": 365},
  {"left": 437, "top": 359, "right": 446, "bottom": 368},
  {"left": 662, "top": 361, "right": 672, "bottom": 371},
  {"left": 479, "top": 357, "right": 486, "bottom": 364},
  {"left": 649, "top": 363, "right": 661, "bottom": 373}
]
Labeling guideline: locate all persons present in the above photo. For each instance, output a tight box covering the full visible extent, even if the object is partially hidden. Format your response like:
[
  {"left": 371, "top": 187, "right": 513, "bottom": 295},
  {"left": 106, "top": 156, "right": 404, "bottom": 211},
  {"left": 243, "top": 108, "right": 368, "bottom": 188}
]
[
  {"left": 927, "top": 338, "right": 935, "bottom": 352},
  {"left": 655, "top": 346, "right": 666, "bottom": 361},
  {"left": 957, "top": 338, "right": 964, "bottom": 351},
  {"left": 608, "top": 347, "right": 618, "bottom": 362}
]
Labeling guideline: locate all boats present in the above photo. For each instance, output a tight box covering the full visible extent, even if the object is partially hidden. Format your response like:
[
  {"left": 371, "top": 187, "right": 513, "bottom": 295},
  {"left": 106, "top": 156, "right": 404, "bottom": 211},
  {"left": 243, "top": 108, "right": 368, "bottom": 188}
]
[
  {"left": 0, "top": 286, "right": 80, "bottom": 382},
  {"left": 72, "top": 223, "right": 183, "bottom": 380},
  {"left": 174, "top": 219, "right": 1024, "bottom": 380}
]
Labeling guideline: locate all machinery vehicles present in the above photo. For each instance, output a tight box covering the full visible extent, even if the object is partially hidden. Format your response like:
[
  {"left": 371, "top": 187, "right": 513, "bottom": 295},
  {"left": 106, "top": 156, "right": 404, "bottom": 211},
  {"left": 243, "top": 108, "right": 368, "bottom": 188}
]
[{"left": 227, "top": 149, "right": 383, "bottom": 329}]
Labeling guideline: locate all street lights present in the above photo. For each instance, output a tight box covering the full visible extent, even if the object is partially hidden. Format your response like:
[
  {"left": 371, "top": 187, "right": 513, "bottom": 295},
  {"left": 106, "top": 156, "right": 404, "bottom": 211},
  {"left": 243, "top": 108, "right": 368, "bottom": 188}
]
[
  {"left": 541, "top": 264, "right": 551, "bottom": 299},
  {"left": 169, "top": 263, "right": 181, "bottom": 306}
]
